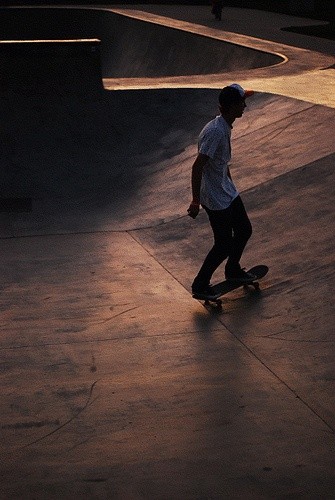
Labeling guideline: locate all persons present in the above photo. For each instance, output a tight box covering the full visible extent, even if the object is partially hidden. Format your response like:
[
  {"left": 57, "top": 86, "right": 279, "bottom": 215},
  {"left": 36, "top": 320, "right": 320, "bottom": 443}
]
[{"left": 187, "top": 83, "right": 256, "bottom": 300}]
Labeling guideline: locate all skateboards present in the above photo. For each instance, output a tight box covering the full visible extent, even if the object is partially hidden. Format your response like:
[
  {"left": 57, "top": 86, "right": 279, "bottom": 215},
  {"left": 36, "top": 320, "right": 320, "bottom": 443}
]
[{"left": 191, "top": 264, "right": 269, "bottom": 307}]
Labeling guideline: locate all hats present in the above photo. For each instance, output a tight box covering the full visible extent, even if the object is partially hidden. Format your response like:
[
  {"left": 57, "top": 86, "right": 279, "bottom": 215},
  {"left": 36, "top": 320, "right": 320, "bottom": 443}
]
[{"left": 219, "top": 84, "right": 254, "bottom": 107}]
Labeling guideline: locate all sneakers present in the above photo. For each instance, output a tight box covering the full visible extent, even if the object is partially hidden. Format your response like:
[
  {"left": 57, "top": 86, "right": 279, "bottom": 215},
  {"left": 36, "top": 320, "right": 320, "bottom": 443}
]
[
  {"left": 191, "top": 283, "right": 221, "bottom": 298},
  {"left": 225, "top": 268, "right": 256, "bottom": 282}
]
